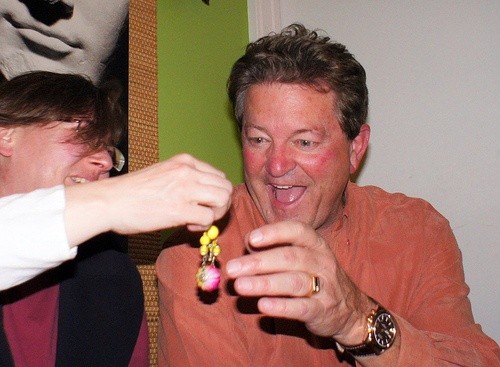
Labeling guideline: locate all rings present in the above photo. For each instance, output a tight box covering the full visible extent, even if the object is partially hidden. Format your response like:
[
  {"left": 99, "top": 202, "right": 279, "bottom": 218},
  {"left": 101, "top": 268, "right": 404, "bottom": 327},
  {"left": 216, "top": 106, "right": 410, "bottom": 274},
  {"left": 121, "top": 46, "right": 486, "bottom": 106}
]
[{"left": 312, "top": 275, "right": 321, "bottom": 295}]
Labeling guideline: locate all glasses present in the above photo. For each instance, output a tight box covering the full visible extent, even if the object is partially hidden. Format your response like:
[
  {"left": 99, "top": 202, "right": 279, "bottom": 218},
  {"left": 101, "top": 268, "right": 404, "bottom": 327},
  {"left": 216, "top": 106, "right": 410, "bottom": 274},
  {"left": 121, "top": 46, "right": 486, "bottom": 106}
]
[{"left": 22, "top": 115, "right": 125, "bottom": 172}]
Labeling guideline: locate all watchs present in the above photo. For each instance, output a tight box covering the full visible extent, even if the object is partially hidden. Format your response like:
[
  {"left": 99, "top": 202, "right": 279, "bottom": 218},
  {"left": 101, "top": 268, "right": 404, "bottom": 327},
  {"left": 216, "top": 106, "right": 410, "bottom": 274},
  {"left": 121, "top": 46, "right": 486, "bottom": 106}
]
[{"left": 335, "top": 294, "right": 397, "bottom": 356}]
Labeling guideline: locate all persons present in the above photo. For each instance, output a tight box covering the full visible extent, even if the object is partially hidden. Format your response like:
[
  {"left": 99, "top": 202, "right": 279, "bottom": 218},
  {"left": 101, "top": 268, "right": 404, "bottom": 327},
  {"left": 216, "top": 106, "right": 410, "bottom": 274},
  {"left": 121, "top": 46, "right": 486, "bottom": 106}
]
[
  {"left": 156, "top": 19, "right": 500, "bottom": 367},
  {"left": 0, "top": 0, "right": 131, "bottom": 86},
  {"left": 0, "top": 70, "right": 233, "bottom": 366}
]
